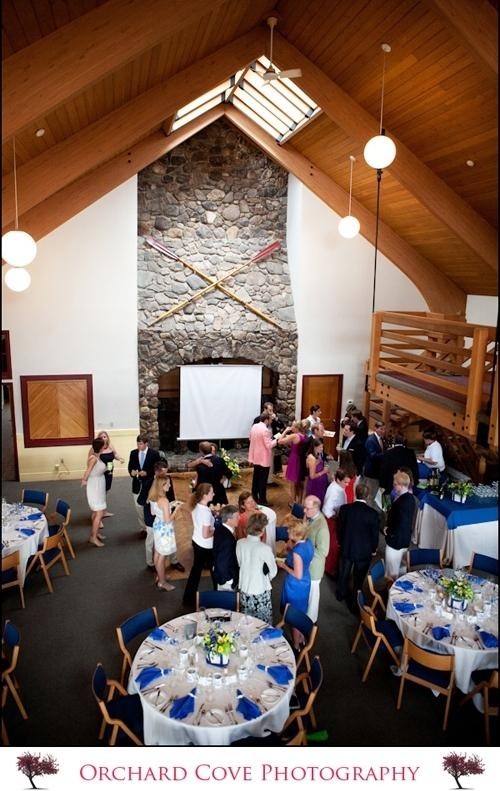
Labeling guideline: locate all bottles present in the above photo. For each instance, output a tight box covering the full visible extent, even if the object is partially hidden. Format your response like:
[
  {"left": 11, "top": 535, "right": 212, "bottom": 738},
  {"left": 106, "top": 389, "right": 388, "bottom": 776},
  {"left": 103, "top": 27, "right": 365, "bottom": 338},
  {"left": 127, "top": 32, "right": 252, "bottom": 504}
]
[
  {"left": 429, "top": 467, "right": 451, "bottom": 501},
  {"left": 384, "top": 430, "right": 409, "bottom": 450}
]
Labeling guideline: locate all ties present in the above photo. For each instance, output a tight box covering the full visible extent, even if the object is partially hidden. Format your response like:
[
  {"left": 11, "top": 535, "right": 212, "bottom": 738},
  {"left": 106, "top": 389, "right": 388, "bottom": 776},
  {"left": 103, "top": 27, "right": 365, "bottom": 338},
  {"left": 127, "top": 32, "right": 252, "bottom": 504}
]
[
  {"left": 233, "top": 531, "right": 237, "bottom": 540},
  {"left": 308, "top": 518, "right": 314, "bottom": 526},
  {"left": 379, "top": 437, "right": 384, "bottom": 453},
  {"left": 140, "top": 452, "right": 145, "bottom": 470}
]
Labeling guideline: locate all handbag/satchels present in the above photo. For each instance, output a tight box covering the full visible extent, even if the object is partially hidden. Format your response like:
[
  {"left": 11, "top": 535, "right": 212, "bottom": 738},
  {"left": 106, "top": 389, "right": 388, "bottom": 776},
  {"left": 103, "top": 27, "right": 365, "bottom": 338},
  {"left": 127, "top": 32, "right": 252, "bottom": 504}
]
[{"left": 262, "top": 561, "right": 269, "bottom": 575}]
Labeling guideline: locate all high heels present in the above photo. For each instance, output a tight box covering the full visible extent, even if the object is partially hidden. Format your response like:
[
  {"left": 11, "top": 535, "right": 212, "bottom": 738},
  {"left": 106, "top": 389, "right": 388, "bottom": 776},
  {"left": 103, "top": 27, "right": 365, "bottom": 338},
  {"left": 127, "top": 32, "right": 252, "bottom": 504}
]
[{"left": 157, "top": 582, "right": 176, "bottom": 592}]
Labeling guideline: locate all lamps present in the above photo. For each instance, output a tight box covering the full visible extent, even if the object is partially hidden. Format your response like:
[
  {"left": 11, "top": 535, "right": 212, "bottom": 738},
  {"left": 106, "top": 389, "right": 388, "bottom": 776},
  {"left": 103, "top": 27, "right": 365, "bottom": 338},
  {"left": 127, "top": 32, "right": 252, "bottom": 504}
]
[
  {"left": 163, "top": 55, "right": 323, "bottom": 145},
  {"left": 5, "top": 268, "right": 30, "bottom": 292},
  {"left": 337, "top": 156, "right": 361, "bottom": 239},
  {"left": 0, "top": 134, "right": 37, "bottom": 268},
  {"left": 362, "top": 42, "right": 397, "bottom": 170}
]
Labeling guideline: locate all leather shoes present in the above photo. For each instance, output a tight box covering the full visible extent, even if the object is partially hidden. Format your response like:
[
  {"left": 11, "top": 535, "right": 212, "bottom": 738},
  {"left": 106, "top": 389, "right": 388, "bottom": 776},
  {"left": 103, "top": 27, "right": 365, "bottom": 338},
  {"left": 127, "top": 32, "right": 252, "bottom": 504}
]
[
  {"left": 95, "top": 533, "right": 106, "bottom": 541},
  {"left": 171, "top": 562, "right": 185, "bottom": 572},
  {"left": 88, "top": 536, "right": 105, "bottom": 547},
  {"left": 267, "top": 481, "right": 279, "bottom": 487},
  {"left": 287, "top": 500, "right": 297, "bottom": 510},
  {"left": 259, "top": 502, "right": 274, "bottom": 508},
  {"left": 102, "top": 511, "right": 115, "bottom": 518},
  {"left": 98, "top": 521, "right": 104, "bottom": 530},
  {"left": 147, "top": 564, "right": 156, "bottom": 572}
]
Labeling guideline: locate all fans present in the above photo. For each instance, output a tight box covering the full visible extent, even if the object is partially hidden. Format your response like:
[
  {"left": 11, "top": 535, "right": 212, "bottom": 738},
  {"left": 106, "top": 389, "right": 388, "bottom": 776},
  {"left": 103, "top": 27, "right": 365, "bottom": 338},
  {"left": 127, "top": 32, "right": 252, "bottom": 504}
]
[{"left": 249, "top": 17, "right": 302, "bottom": 91}]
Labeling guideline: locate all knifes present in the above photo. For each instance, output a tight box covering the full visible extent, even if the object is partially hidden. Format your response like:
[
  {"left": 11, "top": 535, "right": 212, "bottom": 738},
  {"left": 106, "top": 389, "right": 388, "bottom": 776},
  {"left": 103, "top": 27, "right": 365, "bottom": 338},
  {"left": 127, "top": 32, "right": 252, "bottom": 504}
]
[
  {"left": 393, "top": 564, "right": 499, "bottom": 652},
  {"left": 2, "top": 497, "right": 45, "bottom": 552},
  {"left": 137, "top": 607, "right": 295, "bottom": 727}
]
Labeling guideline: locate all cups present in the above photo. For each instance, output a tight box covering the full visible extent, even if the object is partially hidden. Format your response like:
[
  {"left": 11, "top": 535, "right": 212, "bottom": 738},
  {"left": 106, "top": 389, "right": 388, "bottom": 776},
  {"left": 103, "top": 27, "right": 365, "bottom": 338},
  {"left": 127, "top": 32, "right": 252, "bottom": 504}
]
[{"left": 470, "top": 481, "right": 498, "bottom": 505}]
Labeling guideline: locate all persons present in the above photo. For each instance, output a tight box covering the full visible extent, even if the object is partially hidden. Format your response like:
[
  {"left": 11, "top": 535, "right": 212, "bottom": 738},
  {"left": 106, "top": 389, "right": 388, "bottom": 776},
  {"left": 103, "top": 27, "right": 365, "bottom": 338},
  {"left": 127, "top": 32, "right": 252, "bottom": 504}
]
[
  {"left": 81, "top": 437, "right": 109, "bottom": 549},
  {"left": 87, "top": 430, "right": 125, "bottom": 530},
  {"left": 127, "top": 396, "right": 447, "bottom": 652}
]
